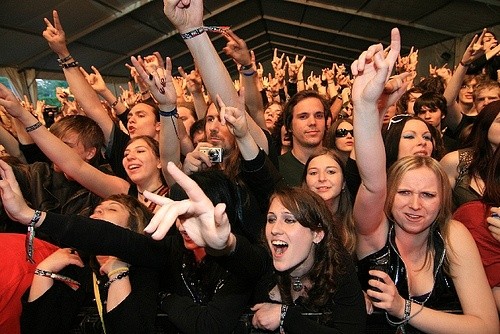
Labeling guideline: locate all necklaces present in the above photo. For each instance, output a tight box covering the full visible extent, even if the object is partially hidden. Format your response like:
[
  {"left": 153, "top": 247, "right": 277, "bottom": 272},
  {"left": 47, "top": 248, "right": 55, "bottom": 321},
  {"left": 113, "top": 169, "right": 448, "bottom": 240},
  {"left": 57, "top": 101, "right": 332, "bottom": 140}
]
[
  {"left": 138, "top": 184, "right": 164, "bottom": 203},
  {"left": 289, "top": 261, "right": 316, "bottom": 292}
]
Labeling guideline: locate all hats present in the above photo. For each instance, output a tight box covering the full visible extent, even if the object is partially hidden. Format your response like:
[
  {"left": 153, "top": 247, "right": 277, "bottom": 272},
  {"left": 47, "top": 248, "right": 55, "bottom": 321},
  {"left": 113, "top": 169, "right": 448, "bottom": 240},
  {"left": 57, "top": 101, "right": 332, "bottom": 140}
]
[
  {"left": 476, "top": 100, "right": 500, "bottom": 125},
  {"left": 189, "top": 118, "right": 205, "bottom": 136}
]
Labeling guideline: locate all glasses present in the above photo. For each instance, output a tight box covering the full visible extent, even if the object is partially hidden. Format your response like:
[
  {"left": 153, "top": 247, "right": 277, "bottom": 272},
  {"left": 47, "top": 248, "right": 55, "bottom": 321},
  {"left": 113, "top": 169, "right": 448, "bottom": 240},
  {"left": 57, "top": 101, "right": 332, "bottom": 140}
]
[
  {"left": 461, "top": 84, "right": 477, "bottom": 89},
  {"left": 387, "top": 114, "right": 414, "bottom": 130},
  {"left": 336, "top": 128, "right": 353, "bottom": 137}
]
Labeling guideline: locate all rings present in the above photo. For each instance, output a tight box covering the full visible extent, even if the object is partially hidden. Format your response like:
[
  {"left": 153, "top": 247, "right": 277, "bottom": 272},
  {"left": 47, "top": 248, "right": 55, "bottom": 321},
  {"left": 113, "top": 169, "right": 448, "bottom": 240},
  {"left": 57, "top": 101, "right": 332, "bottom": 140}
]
[{"left": 70, "top": 247, "right": 76, "bottom": 254}]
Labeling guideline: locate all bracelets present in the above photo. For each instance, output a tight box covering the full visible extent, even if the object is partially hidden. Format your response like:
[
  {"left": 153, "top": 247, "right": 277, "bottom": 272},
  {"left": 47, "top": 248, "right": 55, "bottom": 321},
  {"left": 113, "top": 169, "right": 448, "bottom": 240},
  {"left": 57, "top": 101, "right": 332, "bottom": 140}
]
[
  {"left": 280, "top": 305, "right": 288, "bottom": 334},
  {"left": 431, "top": 72, "right": 436, "bottom": 76},
  {"left": 104, "top": 271, "right": 128, "bottom": 288},
  {"left": 108, "top": 267, "right": 129, "bottom": 276},
  {"left": 59, "top": 61, "right": 79, "bottom": 68},
  {"left": 243, "top": 71, "right": 257, "bottom": 76},
  {"left": 328, "top": 81, "right": 334, "bottom": 84},
  {"left": 110, "top": 99, "right": 119, "bottom": 107},
  {"left": 181, "top": 26, "right": 205, "bottom": 39},
  {"left": 33, "top": 269, "right": 80, "bottom": 291},
  {"left": 338, "top": 95, "right": 343, "bottom": 100},
  {"left": 409, "top": 69, "right": 415, "bottom": 72},
  {"left": 290, "top": 77, "right": 298, "bottom": 81},
  {"left": 58, "top": 54, "right": 72, "bottom": 63},
  {"left": 25, "top": 210, "right": 42, "bottom": 264},
  {"left": 242, "top": 63, "right": 253, "bottom": 71},
  {"left": 142, "top": 89, "right": 149, "bottom": 95},
  {"left": 25, "top": 121, "right": 42, "bottom": 132},
  {"left": 321, "top": 79, "right": 326, "bottom": 86},
  {"left": 160, "top": 108, "right": 180, "bottom": 139},
  {"left": 397, "top": 299, "right": 412, "bottom": 334},
  {"left": 460, "top": 59, "right": 470, "bottom": 67}
]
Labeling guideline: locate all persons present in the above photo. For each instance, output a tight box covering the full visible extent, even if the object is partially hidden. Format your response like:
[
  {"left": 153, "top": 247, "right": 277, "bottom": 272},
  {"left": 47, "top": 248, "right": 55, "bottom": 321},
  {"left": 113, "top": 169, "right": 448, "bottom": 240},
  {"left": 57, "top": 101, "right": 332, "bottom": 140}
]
[{"left": 0, "top": 0, "right": 500, "bottom": 334}]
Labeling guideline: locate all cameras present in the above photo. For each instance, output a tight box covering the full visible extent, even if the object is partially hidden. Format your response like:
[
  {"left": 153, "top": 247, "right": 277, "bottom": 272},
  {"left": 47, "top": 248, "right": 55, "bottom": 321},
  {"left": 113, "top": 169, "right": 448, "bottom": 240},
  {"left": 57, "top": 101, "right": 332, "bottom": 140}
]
[
  {"left": 61, "top": 87, "right": 74, "bottom": 98},
  {"left": 199, "top": 146, "right": 223, "bottom": 163}
]
